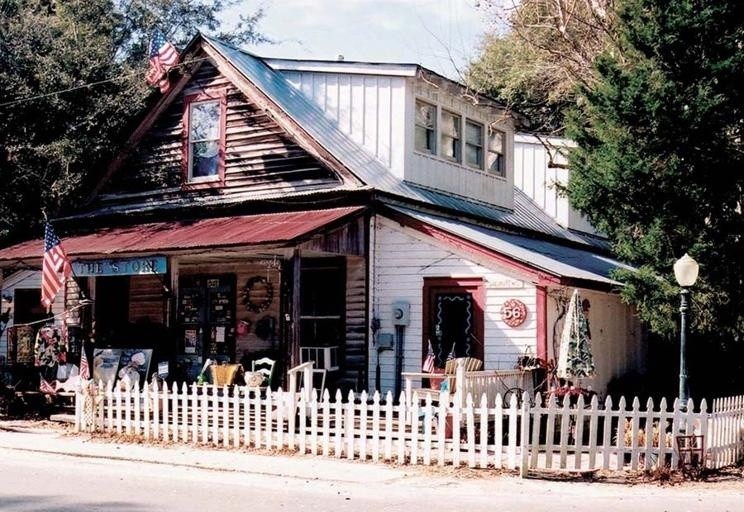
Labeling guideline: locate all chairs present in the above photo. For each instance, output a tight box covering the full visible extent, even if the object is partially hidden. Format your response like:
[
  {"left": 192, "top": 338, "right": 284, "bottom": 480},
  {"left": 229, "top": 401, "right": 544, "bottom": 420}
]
[
  {"left": 404, "top": 355, "right": 484, "bottom": 405},
  {"left": 239, "top": 357, "right": 277, "bottom": 409}
]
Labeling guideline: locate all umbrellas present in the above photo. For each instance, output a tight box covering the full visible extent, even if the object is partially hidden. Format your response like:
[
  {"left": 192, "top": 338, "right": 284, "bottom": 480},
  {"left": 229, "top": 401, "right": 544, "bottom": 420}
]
[{"left": 556, "top": 288, "right": 597, "bottom": 388}]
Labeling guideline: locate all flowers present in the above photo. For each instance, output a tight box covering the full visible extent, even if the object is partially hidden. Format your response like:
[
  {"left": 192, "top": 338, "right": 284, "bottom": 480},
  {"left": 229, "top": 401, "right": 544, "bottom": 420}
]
[{"left": 241, "top": 275, "right": 274, "bottom": 315}]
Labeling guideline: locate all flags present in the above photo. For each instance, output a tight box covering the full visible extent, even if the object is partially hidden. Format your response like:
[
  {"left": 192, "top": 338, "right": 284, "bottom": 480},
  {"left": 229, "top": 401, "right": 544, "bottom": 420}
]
[
  {"left": 422, "top": 343, "right": 436, "bottom": 373},
  {"left": 145, "top": 28, "right": 180, "bottom": 94},
  {"left": 39, "top": 376, "right": 57, "bottom": 396},
  {"left": 80, "top": 346, "right": 91, "bottom": 381},
  {"left": 446, "top": 349, "right": 456, "bottom": 361},
  {"left": 41, "top": 220, "right": 72, "bottom": 307}
]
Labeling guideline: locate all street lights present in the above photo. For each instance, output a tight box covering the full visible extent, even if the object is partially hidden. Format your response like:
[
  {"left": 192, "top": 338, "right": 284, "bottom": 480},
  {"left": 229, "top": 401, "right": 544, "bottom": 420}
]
[{"left": 673, "top": 253, "right": 700, "bottom": 411}]
